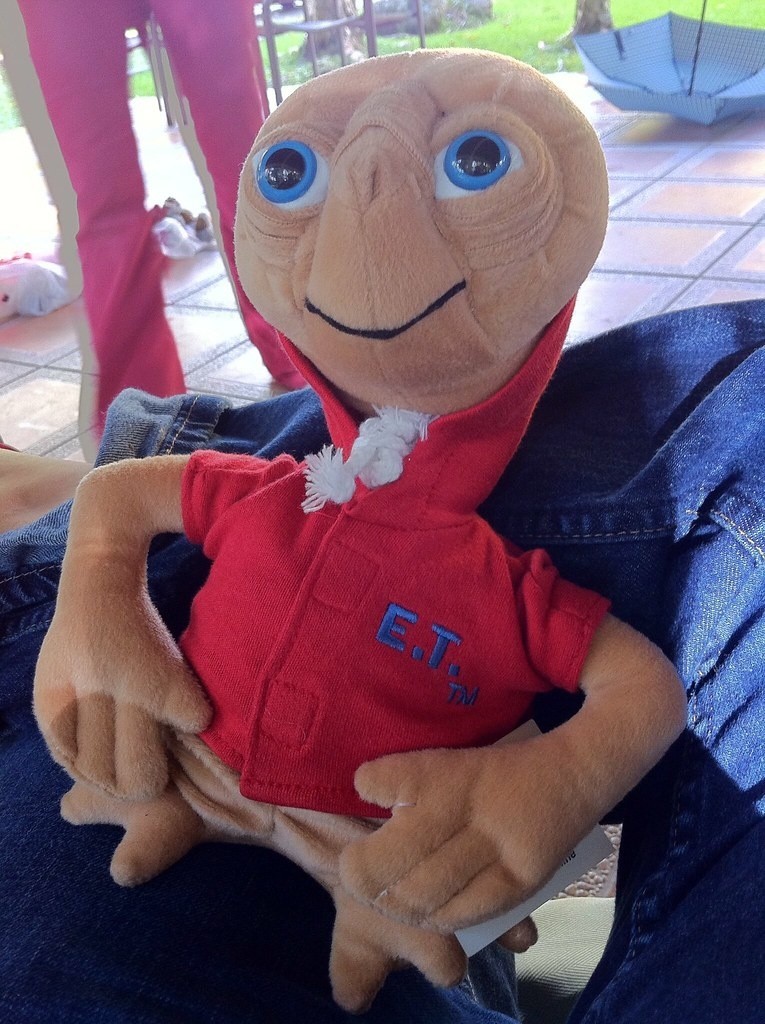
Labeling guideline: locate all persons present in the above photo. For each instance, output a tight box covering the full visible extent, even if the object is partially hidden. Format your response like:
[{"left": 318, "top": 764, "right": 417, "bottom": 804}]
[
  {"left": 0, "top": 0, "right": 308, "bottom": 463},
  {"left": 0, "top": 297, "right": 765, "bottom": 1024}
]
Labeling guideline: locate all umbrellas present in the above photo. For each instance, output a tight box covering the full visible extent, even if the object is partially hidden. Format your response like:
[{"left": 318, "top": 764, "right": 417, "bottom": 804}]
[{"left": 572, "top": 0, "right": 765, "bottom": 128}]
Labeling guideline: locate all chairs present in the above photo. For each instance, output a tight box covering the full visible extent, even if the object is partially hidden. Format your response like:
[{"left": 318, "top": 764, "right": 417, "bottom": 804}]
[{"left": 256, "top": 0, "right": 425, "bottom": 107}]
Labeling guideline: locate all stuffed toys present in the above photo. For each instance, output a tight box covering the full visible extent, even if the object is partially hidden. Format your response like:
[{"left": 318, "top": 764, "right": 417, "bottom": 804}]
[{"left": 32, "top": 48, "right": 685, "bottom": 1016}]
